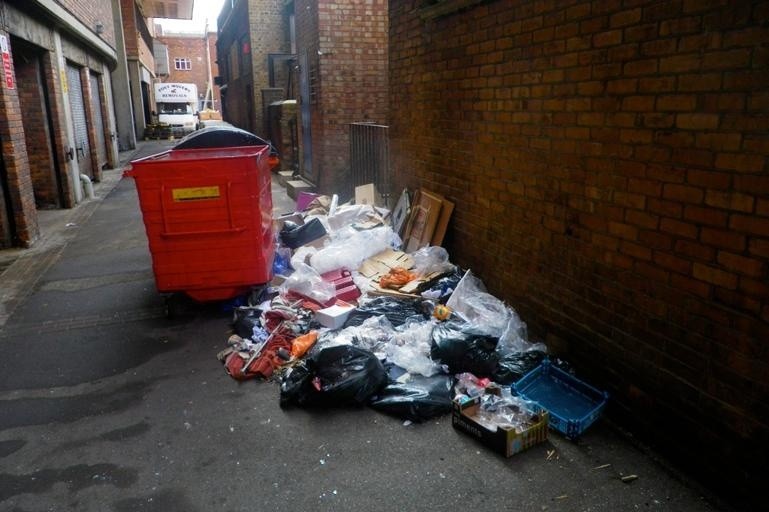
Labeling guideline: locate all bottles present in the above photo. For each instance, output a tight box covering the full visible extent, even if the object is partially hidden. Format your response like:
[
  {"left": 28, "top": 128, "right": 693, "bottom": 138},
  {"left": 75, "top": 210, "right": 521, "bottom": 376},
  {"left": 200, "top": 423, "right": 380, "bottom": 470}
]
[{"left": 419, "top": 299, "right": 464, "bottom": 323}]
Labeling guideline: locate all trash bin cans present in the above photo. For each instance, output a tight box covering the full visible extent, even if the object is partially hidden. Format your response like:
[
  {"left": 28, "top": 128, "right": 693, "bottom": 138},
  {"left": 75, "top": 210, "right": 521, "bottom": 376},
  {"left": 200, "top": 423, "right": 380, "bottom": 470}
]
[{"left": 122, "top": 126, "right": 280, "bottom": 314}]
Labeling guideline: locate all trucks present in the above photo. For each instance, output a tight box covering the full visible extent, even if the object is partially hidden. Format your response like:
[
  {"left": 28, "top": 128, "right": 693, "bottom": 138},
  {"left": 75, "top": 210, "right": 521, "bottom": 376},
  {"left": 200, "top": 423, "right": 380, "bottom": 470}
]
[{"left": 150, "top": 81, "right": 204, "bottom": 136}]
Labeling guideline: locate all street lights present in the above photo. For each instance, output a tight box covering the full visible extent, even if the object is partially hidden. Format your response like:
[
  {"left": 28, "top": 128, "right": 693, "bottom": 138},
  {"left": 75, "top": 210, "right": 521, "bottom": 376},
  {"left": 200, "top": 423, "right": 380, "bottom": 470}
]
[{"left": 204, "top": 18, "right": 214, "bottom": 111}]
